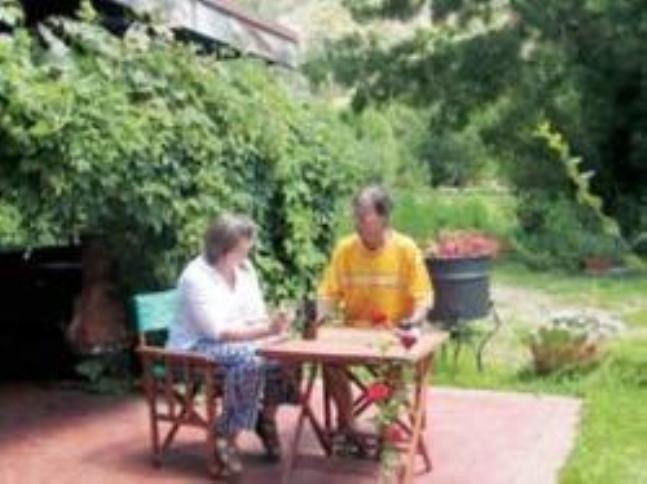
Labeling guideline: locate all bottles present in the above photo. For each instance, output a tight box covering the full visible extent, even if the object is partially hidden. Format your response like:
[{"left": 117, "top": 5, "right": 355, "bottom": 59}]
[{"left": 303, "top": 275, "right": 317, "bottom": 342}]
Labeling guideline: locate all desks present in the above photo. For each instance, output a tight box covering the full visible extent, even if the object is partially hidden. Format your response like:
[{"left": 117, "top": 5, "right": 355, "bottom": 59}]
[{"left": 254, "top": 326, "right": 451, "bottom": 484}]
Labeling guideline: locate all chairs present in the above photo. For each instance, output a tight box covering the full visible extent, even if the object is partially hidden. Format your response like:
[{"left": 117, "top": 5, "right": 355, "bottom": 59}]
[{"left": 126, "top": 287, "right": 223, "bottom": 480}]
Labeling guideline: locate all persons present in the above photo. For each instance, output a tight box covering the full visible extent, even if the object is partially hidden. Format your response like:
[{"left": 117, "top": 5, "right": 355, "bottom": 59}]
[
  {"left": 313, "top": 180, "right": 438, "bottom": 462},
  {"left": 161, "top": 210, "right": 301, "bottom": 480}
]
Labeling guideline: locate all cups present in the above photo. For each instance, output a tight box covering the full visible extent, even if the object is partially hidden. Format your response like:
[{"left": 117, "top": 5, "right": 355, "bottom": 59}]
[
  {"left": 372, "top": 313, "right": 423, "bottom": 354},
  {"left": 277, "top": 297, "right": 298, "bottom": 325}
]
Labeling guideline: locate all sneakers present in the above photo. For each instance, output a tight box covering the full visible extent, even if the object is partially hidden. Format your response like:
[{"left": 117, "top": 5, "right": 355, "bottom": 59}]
[
  {"left": 255, "top": 409, "right": 282, "bottom": 461},
  {"left": 214, "top": 434, "right": 245, "bottom": 475}
]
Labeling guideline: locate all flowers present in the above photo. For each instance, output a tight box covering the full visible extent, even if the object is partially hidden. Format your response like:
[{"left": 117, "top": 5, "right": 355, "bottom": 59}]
[{"left": 418, "top": 224, "right": 502, "bottom": 256}]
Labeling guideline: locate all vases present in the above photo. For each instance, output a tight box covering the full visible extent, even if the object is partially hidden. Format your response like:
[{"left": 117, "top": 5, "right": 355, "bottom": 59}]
[{"left": 422, "top": 257, "right": 491, "bottom": 320}]
